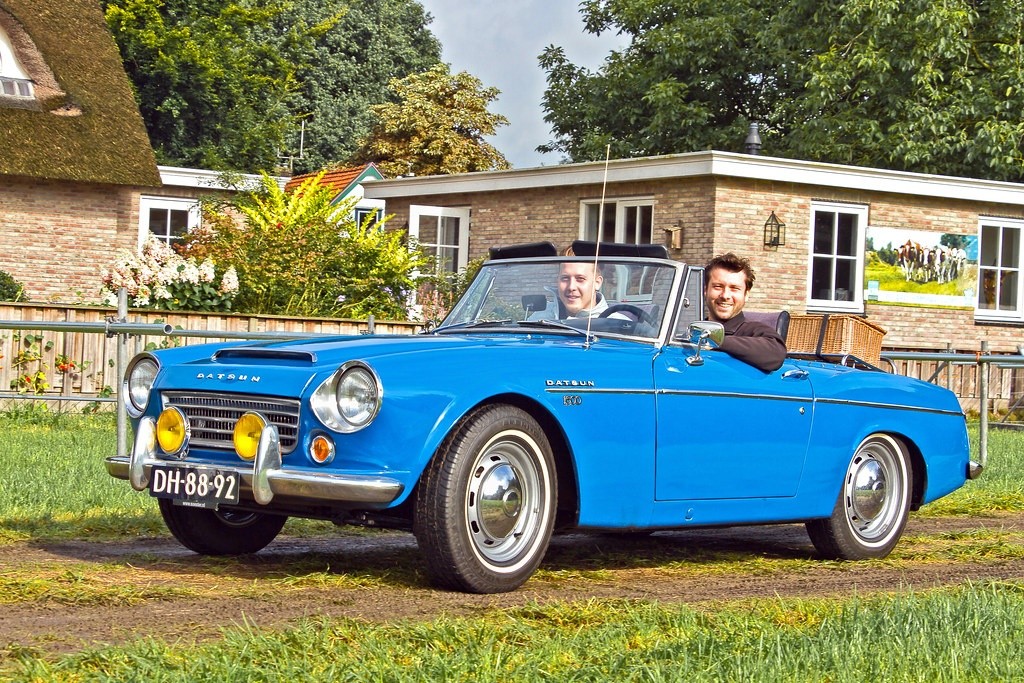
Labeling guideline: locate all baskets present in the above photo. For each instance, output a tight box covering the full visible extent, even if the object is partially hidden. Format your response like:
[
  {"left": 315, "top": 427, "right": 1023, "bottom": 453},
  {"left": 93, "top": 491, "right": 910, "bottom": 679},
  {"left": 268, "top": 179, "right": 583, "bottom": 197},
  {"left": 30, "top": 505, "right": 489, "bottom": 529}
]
[{"left": 784, "top": 313, "right": 887, "bottom": 370}]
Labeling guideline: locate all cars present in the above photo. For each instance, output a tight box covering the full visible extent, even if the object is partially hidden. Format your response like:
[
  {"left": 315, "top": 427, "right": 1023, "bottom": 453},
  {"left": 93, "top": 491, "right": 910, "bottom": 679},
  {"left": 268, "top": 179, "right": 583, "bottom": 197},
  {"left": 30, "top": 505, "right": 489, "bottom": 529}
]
[{"left": 105, "top": 238, "right": 987, "bottom": 594}]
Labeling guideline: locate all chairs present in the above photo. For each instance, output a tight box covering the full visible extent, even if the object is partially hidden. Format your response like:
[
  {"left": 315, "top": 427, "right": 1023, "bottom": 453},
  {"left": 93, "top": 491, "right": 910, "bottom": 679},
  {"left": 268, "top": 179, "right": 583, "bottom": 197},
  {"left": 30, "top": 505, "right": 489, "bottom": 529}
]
[{"left": 742, "top": 310, "right": 791, "bottom": 342}]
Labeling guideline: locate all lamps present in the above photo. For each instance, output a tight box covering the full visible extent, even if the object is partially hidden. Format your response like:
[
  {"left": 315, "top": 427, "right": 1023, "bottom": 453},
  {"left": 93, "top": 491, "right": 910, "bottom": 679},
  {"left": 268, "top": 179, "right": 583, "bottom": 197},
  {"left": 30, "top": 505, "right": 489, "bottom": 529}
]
[{"left": 762, "top": 211, "right": 786, "bottom": 247}]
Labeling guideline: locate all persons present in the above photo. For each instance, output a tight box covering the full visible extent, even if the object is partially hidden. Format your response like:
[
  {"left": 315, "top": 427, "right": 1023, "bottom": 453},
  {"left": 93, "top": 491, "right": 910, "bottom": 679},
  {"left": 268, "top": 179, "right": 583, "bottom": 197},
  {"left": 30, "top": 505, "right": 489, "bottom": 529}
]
[
  {"left": 686, "top": 253, "right": 787, "bottom": 371},
  {"left": 526, "top": 245, "right": 634, "bottom": 322}
]
